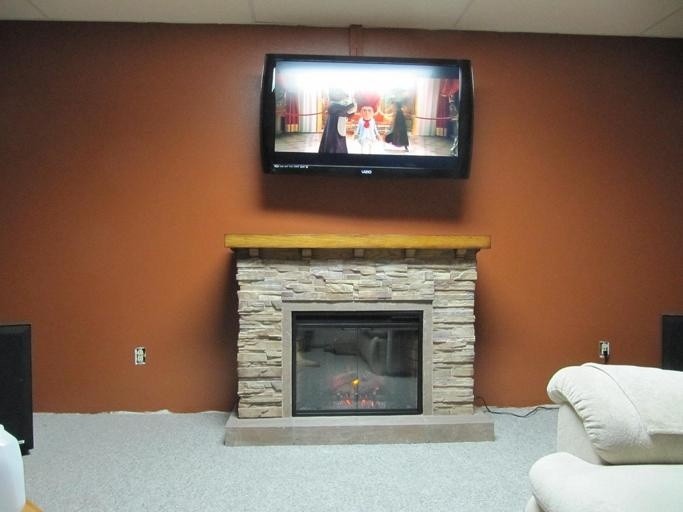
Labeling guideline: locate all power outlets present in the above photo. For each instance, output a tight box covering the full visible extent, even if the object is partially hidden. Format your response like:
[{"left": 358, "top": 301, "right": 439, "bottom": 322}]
[{"left": 600, "top": 341, "right": 610, "bottom": 359}]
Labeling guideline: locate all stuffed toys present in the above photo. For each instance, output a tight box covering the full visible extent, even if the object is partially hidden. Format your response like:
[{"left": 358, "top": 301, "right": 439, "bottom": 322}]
[{"left": 317, "top": 85, "right": 355, "bottom": 155}]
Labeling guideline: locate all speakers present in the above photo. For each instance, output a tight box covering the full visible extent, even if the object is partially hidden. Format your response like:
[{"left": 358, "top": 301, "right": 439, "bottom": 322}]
[
  {"left": 661, "top": 314, "right": 683, "bottom": 371},
  {"left": 0, "top": 323, "right": 34, "bottom": 455}
]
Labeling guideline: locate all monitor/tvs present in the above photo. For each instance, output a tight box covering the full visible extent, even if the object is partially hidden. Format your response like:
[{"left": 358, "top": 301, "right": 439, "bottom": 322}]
[{"left": 260, "top": 52, "right": 474, "bottom": 179}]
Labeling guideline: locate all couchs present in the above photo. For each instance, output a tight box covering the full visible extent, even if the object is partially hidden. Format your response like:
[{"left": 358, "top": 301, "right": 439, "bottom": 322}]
[{"left": 528, "top": 362, "right": 683, "bottom": 511}]
